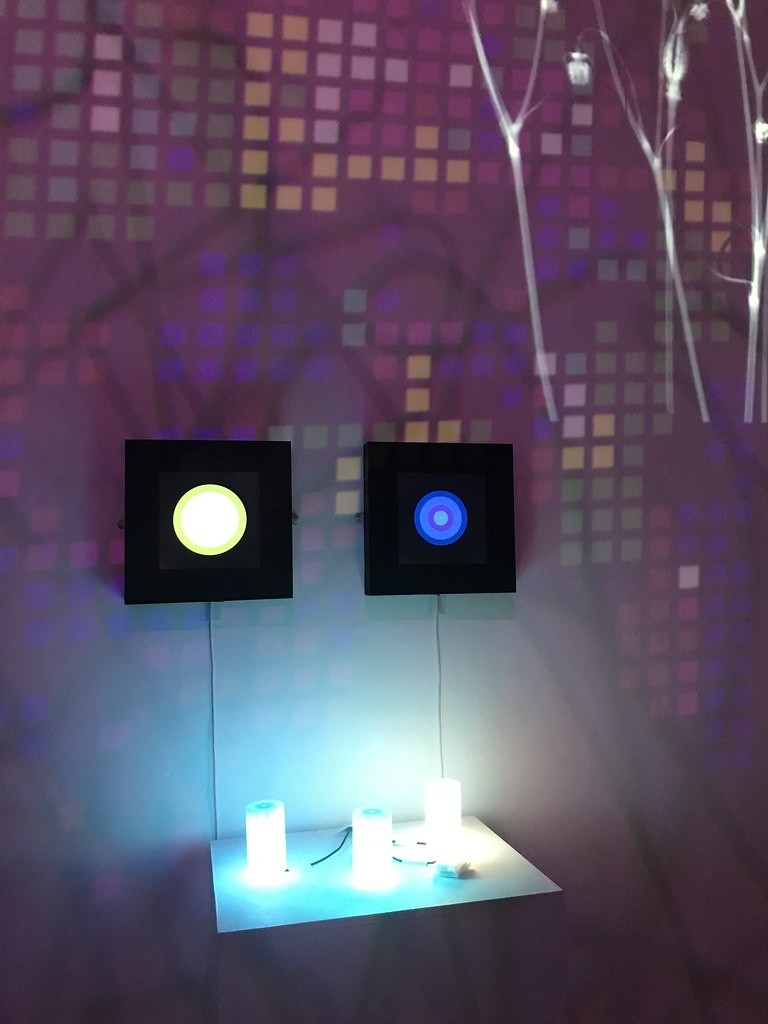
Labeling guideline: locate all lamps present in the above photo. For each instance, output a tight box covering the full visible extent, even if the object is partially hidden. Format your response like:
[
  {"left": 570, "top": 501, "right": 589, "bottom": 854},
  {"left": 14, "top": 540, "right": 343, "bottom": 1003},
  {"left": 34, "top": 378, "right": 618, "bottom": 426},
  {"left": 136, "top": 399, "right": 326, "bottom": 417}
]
[
  {"left": 352, "top": 806, "right": 393, "bottom": 885},
  {"left": 246, "top": 799, "right": 286, "bottom": 876}
]
